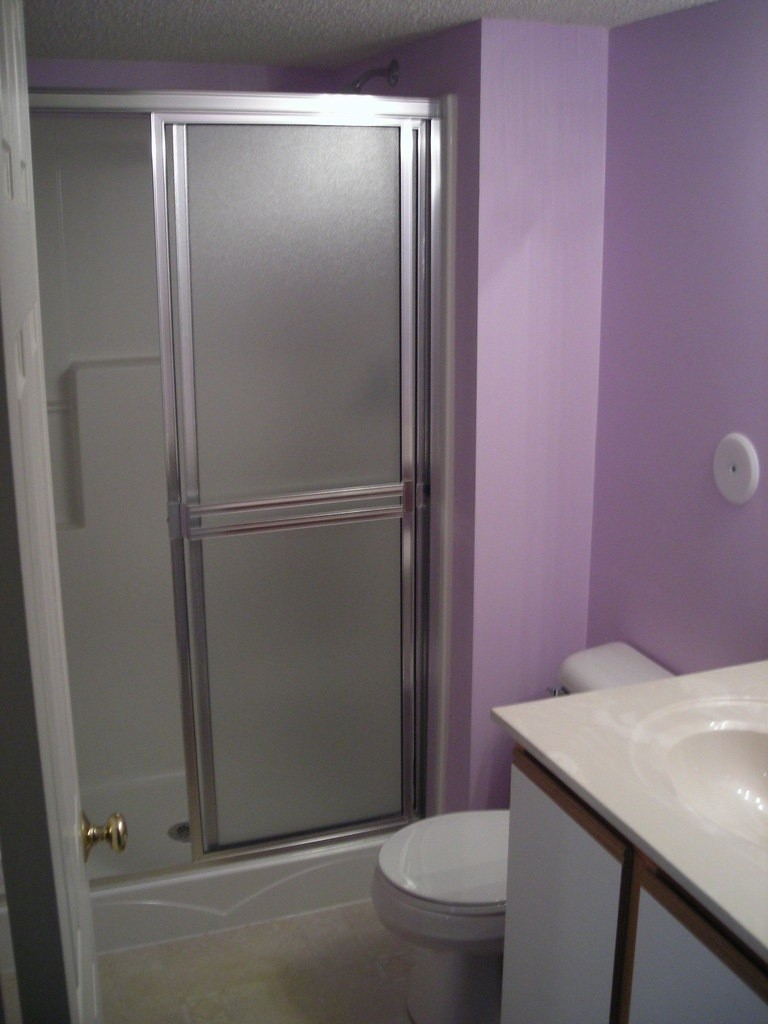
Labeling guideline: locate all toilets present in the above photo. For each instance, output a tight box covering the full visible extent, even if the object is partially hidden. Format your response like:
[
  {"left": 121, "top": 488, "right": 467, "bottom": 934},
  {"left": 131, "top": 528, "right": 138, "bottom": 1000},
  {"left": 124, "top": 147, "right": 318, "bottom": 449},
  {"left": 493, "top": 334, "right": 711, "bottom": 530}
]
[{"left": 370, "top": 638, "right": 678, "bottom": 1024}]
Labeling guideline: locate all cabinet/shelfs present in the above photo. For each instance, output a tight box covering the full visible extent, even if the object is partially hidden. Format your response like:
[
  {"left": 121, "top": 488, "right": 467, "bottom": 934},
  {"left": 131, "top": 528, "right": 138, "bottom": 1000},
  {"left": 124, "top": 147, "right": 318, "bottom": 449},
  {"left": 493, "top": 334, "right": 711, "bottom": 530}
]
[{"left": 490, "top": 746, "right": 768, "bottom": 1024}]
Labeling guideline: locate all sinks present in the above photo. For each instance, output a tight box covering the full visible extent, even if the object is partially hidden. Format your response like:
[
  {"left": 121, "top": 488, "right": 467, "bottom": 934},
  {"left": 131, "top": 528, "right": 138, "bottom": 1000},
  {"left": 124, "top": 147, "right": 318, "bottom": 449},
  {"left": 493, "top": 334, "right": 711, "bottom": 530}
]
[{"left": 630, "top": 691, "right": 768, "bottom": 869}]
